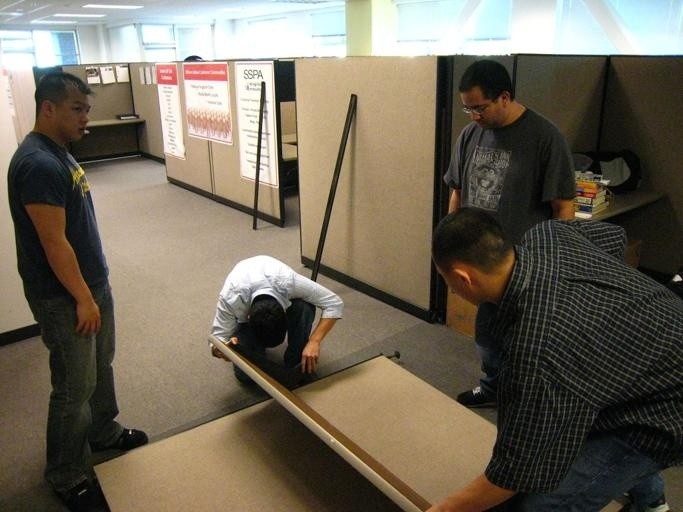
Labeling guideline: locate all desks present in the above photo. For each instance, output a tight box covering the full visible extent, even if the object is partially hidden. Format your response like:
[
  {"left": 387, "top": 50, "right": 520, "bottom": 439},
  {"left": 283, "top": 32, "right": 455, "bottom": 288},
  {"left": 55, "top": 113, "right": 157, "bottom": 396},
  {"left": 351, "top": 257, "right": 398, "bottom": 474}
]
[{"left": 69, "top": 119, "right": 143, "bottom": 166}]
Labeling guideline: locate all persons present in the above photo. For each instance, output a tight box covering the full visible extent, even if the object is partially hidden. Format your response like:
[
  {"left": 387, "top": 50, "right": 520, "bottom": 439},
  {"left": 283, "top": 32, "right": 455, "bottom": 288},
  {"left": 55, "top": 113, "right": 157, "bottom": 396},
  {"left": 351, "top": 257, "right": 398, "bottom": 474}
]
[
  {"left": 4, "top": 71, "right": 148, "bottom": 510},
  {"left": 205, "top": 252, "right": 346, "bottom": 389},
  {"left": 416, "top": 205, "right": 683, "bottom": 512},
  {"left": 440, "top": 60, "right": 579, "bottom": 409}
]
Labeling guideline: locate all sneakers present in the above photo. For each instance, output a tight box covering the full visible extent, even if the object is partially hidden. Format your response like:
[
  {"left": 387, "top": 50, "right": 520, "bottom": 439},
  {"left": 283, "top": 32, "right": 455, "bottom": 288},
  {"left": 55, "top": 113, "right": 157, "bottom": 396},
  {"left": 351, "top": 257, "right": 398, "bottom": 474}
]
[
  {"left": 54, "top": 477, "right": 109, "bottom": 511},
  {"left": 457, "top": 386, "right": 499, "bottom": 408},
  {"left": 89, "top": 428, "right": 148, "bottom": 454},
  {"left": 622, "top": 491, "right": 670, "bottom": 512}
]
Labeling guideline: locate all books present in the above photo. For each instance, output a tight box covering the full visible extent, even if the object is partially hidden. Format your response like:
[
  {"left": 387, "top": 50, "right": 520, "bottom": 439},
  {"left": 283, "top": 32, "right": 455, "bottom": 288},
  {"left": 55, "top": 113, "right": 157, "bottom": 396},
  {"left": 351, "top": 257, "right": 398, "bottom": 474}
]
[{"left": 575, "top": 179, "right": 611, "bottom": 221}]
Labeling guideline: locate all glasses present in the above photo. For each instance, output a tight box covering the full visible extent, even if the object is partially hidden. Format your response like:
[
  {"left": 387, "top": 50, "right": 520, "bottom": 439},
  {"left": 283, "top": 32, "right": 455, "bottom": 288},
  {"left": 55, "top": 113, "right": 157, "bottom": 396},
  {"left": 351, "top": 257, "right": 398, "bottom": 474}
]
[{"left": 461, "top": 96, "right": 500, "bottom": 115}]
